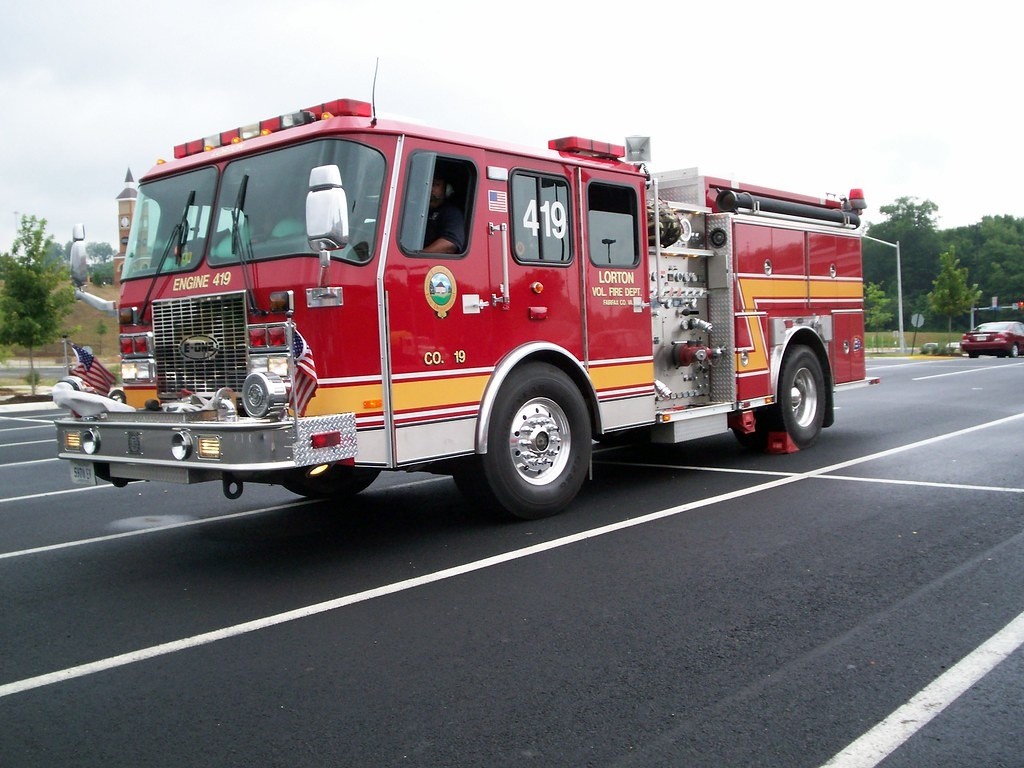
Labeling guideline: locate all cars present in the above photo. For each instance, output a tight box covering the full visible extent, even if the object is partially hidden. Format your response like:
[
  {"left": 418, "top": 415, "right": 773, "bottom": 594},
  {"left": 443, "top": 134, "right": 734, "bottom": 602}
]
[{"left": 960, "top": 321, "right": 1024, "bottom": 359}]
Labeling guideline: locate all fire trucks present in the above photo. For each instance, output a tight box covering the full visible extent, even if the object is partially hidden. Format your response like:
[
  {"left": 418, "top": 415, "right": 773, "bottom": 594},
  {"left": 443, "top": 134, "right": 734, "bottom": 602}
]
[{"left": 47, "top": 57, "right": 884, "bottom": 520}]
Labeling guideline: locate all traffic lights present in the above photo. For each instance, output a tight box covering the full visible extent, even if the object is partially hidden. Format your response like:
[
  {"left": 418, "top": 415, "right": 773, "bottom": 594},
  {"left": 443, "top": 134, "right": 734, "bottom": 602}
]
[{"left": 1018, "top": 301, "right": 1024, "bottom": 308}]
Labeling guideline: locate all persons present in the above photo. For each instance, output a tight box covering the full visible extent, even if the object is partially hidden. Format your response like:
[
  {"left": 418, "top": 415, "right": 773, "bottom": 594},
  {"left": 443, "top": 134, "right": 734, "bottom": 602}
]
[{"left": 422, "top": 166, "right": 467, "bottom": 253}]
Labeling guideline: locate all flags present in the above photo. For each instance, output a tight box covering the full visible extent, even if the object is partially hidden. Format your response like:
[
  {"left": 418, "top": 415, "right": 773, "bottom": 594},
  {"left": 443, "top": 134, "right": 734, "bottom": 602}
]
[{"left": 68, "top": 341, "right": 117, "bottom": 397}]
